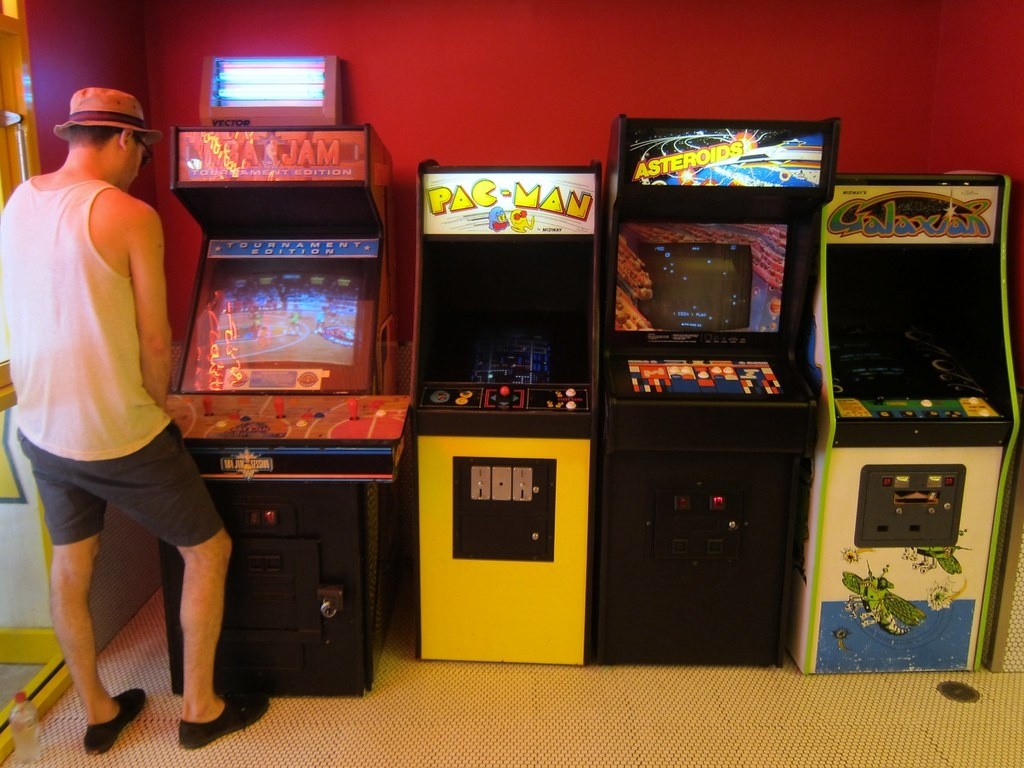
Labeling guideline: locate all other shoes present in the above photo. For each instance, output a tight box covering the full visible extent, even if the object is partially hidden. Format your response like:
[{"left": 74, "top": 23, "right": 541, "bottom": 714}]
[
  {"left": 83, "top": 688, "right": 148, "bottom": 755},
  {"left": 177, "top": 693, "right": 271, "bottom": 749}
]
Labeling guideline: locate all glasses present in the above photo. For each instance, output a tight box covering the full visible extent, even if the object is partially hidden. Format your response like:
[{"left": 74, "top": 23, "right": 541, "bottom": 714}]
[{"left": 115, "top": 128, "right": 152, "bottom": 168}]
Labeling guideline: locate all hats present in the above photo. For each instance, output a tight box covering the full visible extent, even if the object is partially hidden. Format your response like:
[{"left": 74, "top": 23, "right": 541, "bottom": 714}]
[{"left": 53, "top": 87, "right": 162, "bottom": 145}]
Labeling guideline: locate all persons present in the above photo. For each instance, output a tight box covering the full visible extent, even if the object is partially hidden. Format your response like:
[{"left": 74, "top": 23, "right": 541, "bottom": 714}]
[{"left": 0, "top": 88, "right": 271, "bottom": 756}]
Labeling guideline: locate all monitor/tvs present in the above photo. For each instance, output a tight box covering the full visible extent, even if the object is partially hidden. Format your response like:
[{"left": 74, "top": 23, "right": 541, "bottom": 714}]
[
  {"left": 430, "top": 309, "right": 592, "bottom": 385},
  {"left": 180, "top": 257, "right": 377, "bottom": 395},
  {"left": 830, "top": 330, "right": 981, "bottom": 398},
  {"left": 613, "top": 217, "right": 796, "bottom": 357}
]
